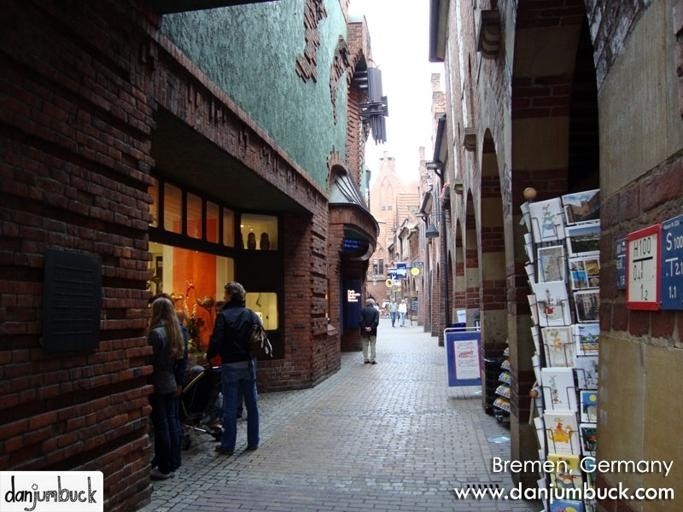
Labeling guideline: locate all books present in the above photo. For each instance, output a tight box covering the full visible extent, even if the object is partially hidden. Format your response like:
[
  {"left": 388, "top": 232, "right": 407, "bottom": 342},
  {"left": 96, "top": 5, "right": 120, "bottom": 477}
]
[{"left": 518, "top": 186, "right": 607, "bottom": 512}]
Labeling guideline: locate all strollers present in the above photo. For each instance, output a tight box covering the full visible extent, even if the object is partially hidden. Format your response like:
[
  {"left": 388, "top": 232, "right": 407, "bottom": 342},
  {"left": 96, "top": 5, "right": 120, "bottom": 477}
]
[{"left": 181, "top": 365, "right": 226, "bottom": 451}]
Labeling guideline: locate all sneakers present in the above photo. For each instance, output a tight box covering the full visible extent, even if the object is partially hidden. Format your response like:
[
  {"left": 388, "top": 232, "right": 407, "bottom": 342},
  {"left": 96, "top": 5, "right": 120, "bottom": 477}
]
[
  {"left": 148, "top": 463, "right": 177, "bottom": 482},
  {"left": 207, "top": 447, "right": 224, "bottom": 458},
  {"left": 364, "top": 360, "right": 377, "bottom": 365}
]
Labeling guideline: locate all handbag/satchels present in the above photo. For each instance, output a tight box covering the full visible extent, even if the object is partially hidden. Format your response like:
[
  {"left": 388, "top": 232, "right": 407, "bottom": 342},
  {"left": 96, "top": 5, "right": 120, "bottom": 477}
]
[{"left": 251, "top": 324, "right": 273, "bottom": 362}]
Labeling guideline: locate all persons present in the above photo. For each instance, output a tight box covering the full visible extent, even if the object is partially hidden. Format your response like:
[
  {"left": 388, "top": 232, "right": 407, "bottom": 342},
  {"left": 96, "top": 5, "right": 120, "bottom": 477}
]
[
  {"left": 396, "top": 299, "right": 408, "bottom": 326},
  {"left": 389, "top": 296, "right": 398, "bottom": 327},
  {"left": 152, "top": 293, "right": 188, "bottom": 472},
  {"left": 150, "top": 297, "right": 178, "bottom": 478},
  {"left": 212, "top": 297, "right": 248, "bottom": 420},
  {"left": 205, "top": 281, "right": 263, "bottom": 455},
  {"left": 359, "top": 298, "right": 380, "bottom": 365}
]
